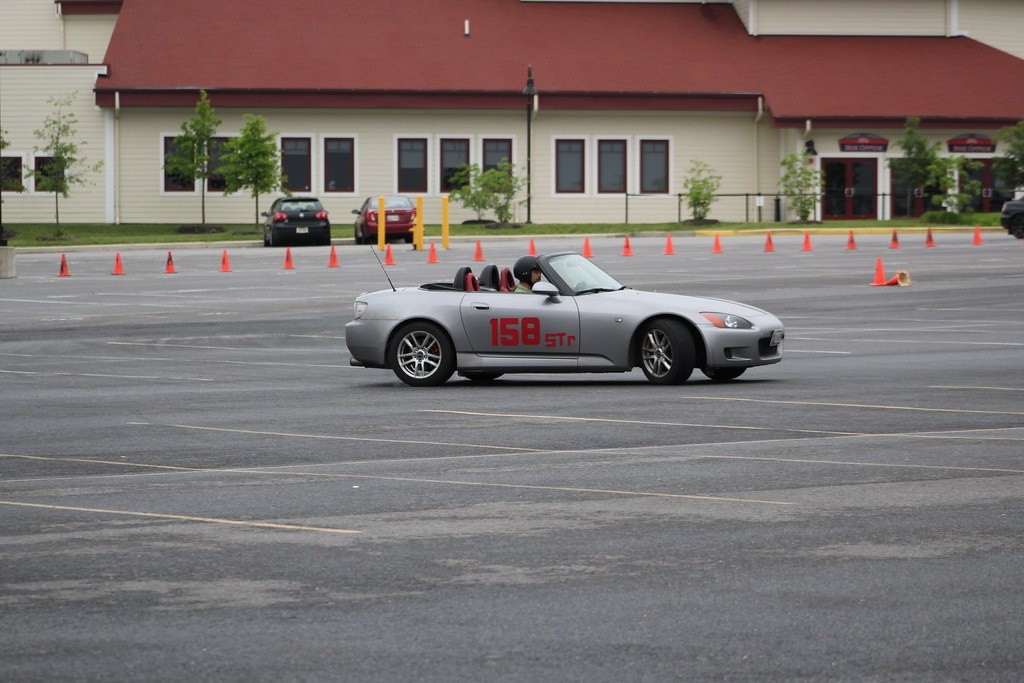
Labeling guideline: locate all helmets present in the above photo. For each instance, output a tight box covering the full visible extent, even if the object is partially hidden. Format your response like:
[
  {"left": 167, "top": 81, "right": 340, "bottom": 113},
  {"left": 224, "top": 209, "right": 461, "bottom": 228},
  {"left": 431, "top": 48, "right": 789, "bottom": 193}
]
[{"left": 514, "top": 256, "right": 541, "bottom": 286}]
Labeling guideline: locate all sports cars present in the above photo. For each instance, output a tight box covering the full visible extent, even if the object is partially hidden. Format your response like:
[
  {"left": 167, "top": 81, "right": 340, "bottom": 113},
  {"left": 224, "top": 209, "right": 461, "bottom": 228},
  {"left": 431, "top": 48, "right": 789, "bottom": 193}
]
[{"left": 344, "top": 250, "right": 787, "bottom": 387}]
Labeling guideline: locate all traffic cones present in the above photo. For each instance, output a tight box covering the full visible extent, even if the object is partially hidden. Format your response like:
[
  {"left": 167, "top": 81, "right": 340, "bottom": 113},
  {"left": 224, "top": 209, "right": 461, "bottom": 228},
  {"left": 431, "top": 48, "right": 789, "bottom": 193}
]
[
  {"left": 800, "top": 231, "right": 814, "bottom": 251},
  {"left": 56, "top": 253, "right": 72, "bottom": 275},
  {"left": 282, "top": 246, "right": 297, "bottom": 270},
  {"left": 582, "top": 235, "right": 595, "bottom": 258},
  {"left": 162, "top": 251, "right": 179, "bottom": 274},
  {"left": 111, "top": 252, "right": 126, "bottom": 275},
  {"left": 620, "top": 232, "right": 637, "bottom": 256},
  {"left": 761, "top": 230, "right": 776, "bottom": 252},
  {"left": 886, "top": 270, "right": 911, "bottom": 288},
  {"left": 972, "top": 226, "right": 983, "bottom": 246},
  {"left": 327, "top": 246, "right": 341, "bottom": 267},
  {"left": 711, "top": 233, "right": 723, "bottom": 253},
  {"left": 889, "top": 230, "right": 900, "bottom": 249},
  {"left": 472, "top": 239, "right": 486, "bottom": 262},
  {"left": 924, "top": 227, "right": 936, "bottom": 247},
  {"left": 869, "top": 256, "right": 887, "bottom": 285},
  {"left": 426, "top": 242, "right": 442, "bottom": 264},
  {"left": 662, "top": 233, "right": 676, "bottom": 255},
  {"left": 845, "top": 229, "right": 857, "bottom": 250},
  {"left": 218, "top": 249, "right": 233, "bottom": 273},
  {"left": 527, "top": 239, "right": 537, "bottom": 256},
  {"left": 382, "top": 244, "right": 398, "bottom": 265}
]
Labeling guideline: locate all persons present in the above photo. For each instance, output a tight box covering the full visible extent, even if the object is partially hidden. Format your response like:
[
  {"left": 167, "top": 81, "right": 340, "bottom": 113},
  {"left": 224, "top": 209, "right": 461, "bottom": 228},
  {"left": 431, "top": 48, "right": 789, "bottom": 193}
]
[{"left": 513, "top": 256, "right": 542, "bottom": 292}]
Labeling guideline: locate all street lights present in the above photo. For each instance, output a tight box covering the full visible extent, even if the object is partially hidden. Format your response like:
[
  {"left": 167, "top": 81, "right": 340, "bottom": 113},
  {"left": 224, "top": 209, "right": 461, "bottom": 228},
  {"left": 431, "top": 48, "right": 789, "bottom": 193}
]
[{"left": 522, "top": 64, "right": 537, "bottom": 224}]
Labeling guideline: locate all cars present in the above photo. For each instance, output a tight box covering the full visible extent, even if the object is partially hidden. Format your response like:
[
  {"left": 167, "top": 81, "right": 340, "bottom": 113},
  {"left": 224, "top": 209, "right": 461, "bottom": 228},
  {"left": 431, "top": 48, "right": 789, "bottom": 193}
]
[
  {"left": 1000, "top": 196, "right": 1024, "bottom": 240},
  {"left": 351, "top": 195, "right": 417, "bottom": 244},
  {"left": 260, "top": 197, "right": 331, "bottom": 247}
]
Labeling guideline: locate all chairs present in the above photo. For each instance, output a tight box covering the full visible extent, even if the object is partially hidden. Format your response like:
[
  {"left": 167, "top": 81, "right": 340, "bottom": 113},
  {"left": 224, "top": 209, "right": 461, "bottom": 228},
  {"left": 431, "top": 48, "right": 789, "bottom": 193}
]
[
  {"left": 464, "top": 272, "right": 481, "bottom": 292},
  {"left": 499, "top": 268, "right": 516, "bottom": 292}
]
